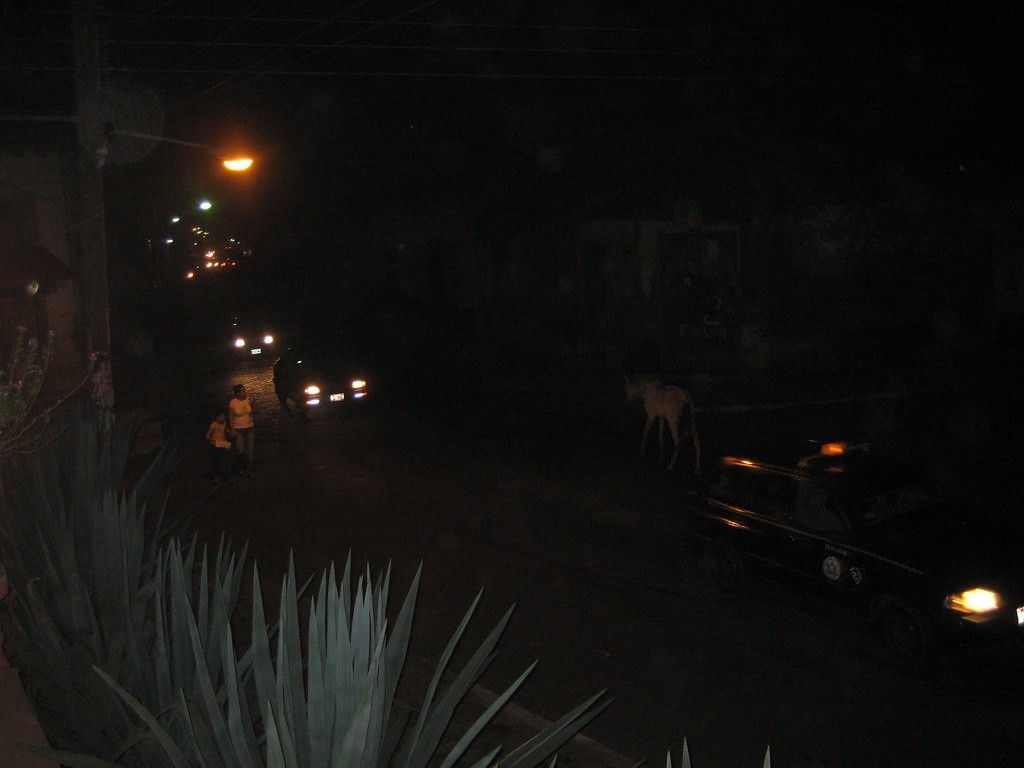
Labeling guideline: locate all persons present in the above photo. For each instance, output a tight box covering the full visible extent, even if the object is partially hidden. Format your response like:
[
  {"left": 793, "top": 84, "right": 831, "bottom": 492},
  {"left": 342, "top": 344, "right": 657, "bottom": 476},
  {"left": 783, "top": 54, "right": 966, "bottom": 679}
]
[
  {"left": 204, "top": 409, "right": 229, "bottom": 479},
  {"left": 226, "top": 385, "right": 258, "bottom": 478}
]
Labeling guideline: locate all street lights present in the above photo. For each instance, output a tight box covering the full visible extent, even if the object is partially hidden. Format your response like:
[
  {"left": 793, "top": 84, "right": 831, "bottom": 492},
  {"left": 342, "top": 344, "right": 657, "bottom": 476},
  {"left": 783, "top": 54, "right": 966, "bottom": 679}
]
[{"left": 79, "top": 128, "right": 256, "bottom": 642}]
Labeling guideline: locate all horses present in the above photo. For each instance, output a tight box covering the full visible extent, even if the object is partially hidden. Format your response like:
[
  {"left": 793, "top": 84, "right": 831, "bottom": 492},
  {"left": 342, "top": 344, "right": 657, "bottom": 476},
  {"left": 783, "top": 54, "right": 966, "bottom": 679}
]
[{"left": 624, "top": 376, "right": 701, "bottom": 475}]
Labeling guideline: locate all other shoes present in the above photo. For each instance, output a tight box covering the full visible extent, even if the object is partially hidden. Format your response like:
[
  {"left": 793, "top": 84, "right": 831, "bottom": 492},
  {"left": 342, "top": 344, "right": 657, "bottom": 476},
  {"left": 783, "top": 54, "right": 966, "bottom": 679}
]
[
  {"left": 240, "top": 470, "right": 244, "bottom": 476},
  {"left": 248, "top": 473, "right": 256, "bottom": 479}
]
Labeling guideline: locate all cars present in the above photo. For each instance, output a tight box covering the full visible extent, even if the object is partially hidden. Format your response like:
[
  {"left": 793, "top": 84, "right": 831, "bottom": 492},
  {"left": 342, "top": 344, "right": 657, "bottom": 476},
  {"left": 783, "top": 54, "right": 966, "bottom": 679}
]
[
  {"left": 272, "top": 342, "right": 383, "bottom": 429},
  {"left": 682, "top": 432, "right": 1024, "bottom": 675}
]
